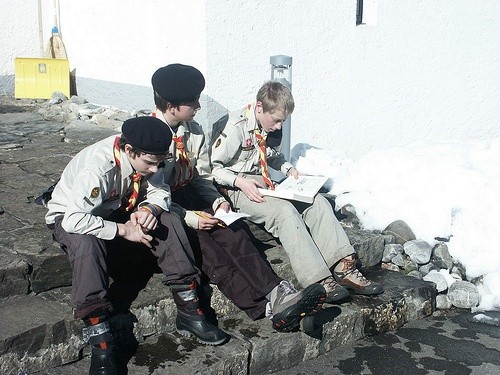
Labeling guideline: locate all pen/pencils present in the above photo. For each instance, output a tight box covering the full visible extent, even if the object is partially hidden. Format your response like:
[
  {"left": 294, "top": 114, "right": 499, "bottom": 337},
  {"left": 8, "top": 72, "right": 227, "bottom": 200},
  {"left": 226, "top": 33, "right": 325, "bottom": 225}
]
[{"left": 193, "top": 211, "right": 226, "bottom": 228}]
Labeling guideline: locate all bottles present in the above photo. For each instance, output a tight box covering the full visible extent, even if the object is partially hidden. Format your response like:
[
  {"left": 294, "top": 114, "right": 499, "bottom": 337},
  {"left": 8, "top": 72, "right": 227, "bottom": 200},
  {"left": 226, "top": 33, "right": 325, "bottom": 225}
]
[{"left": 43, "top": 25, "right": 66, "bottom": 59}]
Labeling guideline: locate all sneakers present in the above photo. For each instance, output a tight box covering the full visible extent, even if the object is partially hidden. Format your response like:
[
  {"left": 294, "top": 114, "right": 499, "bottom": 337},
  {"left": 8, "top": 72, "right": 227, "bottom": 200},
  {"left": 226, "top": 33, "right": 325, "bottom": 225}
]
[
  {"left": 264, "top": 278, "right": 327, "bottom": 335},
  {"left": 318, "top": 275, "right": 351, "bottom": 303},
  {"left": 330, "top": 254, "right": 385, "bottom": 296}
]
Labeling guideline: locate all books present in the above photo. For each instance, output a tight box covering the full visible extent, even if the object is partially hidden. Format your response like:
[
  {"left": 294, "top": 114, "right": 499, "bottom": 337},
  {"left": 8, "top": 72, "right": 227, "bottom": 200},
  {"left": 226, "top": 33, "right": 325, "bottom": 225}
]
[{"left": 257, "top": 174, "right": 328, "bottom": 204}]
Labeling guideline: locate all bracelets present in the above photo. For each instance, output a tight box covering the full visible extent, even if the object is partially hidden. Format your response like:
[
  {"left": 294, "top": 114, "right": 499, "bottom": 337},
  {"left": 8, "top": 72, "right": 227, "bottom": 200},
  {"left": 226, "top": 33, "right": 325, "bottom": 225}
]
[{"left": 142, "top": 204, "right": 158, "bottom": 217}]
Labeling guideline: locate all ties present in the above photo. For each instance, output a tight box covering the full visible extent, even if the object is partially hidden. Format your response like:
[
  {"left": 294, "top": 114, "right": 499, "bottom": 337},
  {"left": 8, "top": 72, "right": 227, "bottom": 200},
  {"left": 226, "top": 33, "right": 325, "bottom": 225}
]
[
  {"left": 114, "top": 136, "right": 147, "bottom": 212},
  {"left": 152, "top": 111, "right": 194, "bottom": 188},
  {"left": 246, "top": 104, "right": 276, "bottom": 191}
]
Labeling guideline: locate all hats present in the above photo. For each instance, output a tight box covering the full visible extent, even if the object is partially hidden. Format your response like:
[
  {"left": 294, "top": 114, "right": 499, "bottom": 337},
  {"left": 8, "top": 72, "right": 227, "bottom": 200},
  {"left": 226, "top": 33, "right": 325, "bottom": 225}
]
[
  {"left": 122, "top": 116, "right": 172, "bottom": 156},
  {"left": 151, "top": 63, "right": 205, "bottom": 105}
]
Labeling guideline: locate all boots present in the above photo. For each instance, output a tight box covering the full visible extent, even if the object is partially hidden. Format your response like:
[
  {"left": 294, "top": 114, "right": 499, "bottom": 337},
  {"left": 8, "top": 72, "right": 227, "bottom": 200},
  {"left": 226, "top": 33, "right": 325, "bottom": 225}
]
[
  {"left": 81, "top": 310, "right": 124, "bottom": 375},
  {"left": 169, "top": 278, "right": 229, "bottom": 346}
]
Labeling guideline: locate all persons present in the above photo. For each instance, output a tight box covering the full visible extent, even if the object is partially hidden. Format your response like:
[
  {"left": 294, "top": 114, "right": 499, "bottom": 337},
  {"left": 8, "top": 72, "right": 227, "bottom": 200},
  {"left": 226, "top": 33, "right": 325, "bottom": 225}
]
[
  {"left": 43, "top": 116, "right": 228, "bottom": 373},
  {"left": 132, "top": 64, "right": 326, "bottom": 333},
  {"left": 206, "top": 81, "right": 383, "bottom": 302}
]
